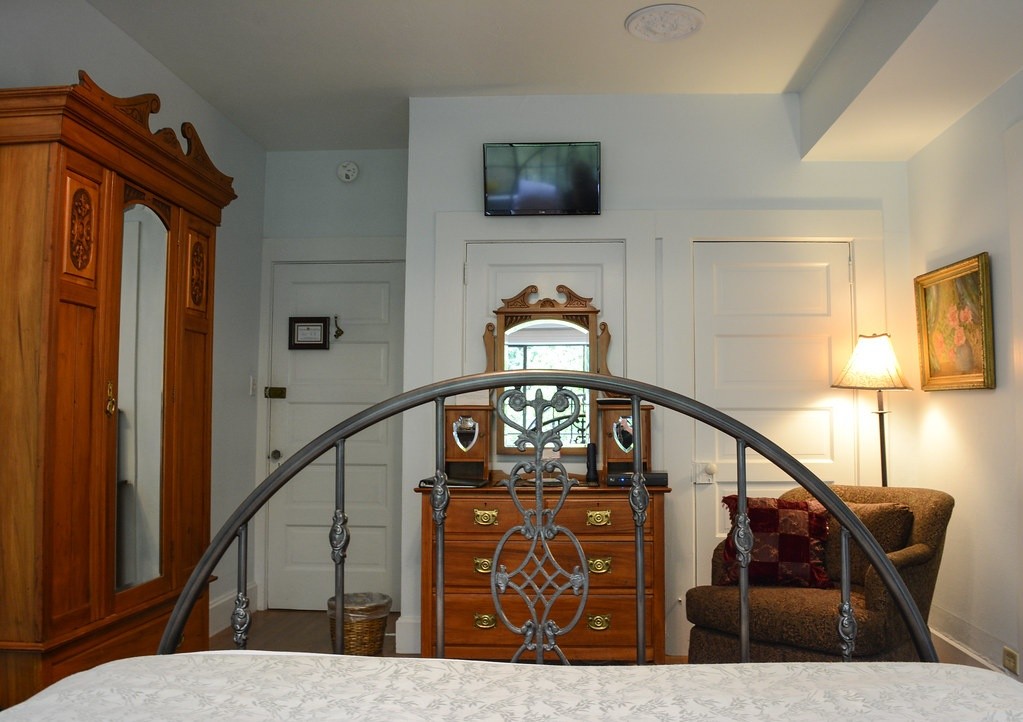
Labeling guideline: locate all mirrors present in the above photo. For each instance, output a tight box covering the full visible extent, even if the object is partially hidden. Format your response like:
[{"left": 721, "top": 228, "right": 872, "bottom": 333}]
[
  {"left": 115, "top": 203, "right": 169, "bottom": 596},
  {"left": 492, "top": 282, "right": 600, "bottom": 456},
  {"left": 613, "top": 416, "right": 637, "bottom": 453},
  {"left": 452, "top": 416, "right": 478, "bottom": 452}
]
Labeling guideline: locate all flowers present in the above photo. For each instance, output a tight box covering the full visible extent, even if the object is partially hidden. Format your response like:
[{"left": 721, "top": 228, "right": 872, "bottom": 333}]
[{"left": 933, "top": 302, "right": 972, "bottom": 361}]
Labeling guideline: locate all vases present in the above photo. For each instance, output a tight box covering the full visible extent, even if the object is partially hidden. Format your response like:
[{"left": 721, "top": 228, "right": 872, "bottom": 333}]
[{"left": 955, "top": 338, "right": 974, "bottom": 373}]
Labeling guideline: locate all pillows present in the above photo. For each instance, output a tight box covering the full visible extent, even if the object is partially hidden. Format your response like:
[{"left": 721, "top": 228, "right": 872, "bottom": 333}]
[
  {"left": 717, "top": 495, "right": 835, "bottom": 590},
  {"left": 803, "top": 498, "right": 914, "bottom": 588}
]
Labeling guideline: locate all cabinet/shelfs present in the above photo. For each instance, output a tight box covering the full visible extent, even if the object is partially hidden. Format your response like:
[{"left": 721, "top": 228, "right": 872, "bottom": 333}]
[
  {"left": 0, "top": 70, "right": 239, "bottom": 714},
  {"left": 414, "top": 482, "right": 672, "bottom": 667}
]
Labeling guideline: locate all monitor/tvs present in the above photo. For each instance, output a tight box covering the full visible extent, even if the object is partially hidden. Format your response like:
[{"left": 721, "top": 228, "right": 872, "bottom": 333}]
[{"left": 483, "top": 142, "right": 602, "bottom": 216}]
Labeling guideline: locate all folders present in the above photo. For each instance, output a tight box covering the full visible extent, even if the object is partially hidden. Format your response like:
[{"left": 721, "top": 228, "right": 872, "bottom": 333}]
[{"left": 420, "top": 476, "right": 488, "bottom": 489}]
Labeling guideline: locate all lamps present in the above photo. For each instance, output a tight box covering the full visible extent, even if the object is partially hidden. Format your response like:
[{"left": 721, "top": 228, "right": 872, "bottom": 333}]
[
  {"left": 624, "top": 4, "right": 706, "bottom": 43},
  {"left": 830, "top": 334, "right": 914, "bottom": 488}
]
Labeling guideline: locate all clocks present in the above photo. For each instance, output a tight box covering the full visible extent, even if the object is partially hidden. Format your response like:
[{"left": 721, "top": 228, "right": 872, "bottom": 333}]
[{"left": 336, "top": 160, "right": 360, "bottom": 183}]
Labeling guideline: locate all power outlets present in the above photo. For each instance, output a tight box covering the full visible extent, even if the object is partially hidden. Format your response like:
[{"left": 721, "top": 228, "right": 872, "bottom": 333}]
[{"left": 1002, "top": 645, "right": 1019, "bottom": 676}]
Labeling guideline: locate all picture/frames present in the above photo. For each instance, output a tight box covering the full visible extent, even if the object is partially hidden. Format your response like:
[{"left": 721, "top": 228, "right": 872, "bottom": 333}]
[
  {"left": 288, "top": 316, "right": 330, "bottom": 351},
  {"left": 913, "top": 252, "right": 997, "bottom": 391}
]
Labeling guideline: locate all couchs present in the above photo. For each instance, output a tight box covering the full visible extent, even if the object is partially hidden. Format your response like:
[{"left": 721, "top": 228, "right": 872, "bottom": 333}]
[{"left": 685, "top": 484, "right": 955, "bottom": 663}]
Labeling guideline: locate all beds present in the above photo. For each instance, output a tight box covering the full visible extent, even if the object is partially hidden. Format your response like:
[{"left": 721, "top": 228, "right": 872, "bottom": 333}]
[{"left": 1, "top": 650, "right": 1023, "bottom": 721}]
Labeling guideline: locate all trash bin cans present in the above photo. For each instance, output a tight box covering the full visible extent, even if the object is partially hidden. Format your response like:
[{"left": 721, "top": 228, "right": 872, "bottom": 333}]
[{"left": 329, "top": 593, "right": 393, "bottom": 656}]
